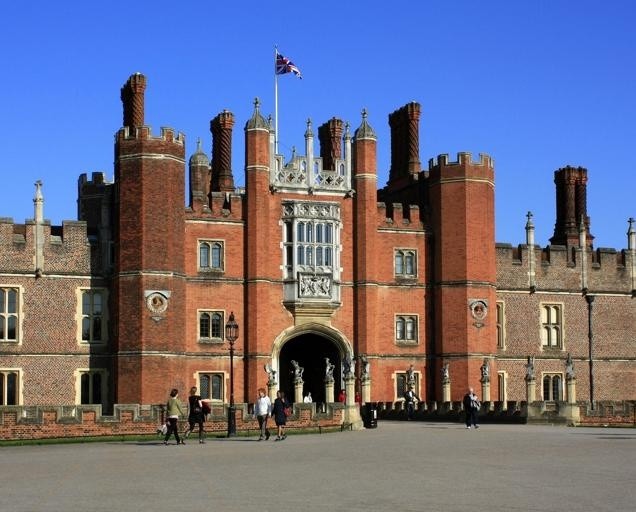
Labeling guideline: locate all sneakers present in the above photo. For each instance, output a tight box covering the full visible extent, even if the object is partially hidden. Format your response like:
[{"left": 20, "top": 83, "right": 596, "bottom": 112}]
[
  {"left": 467, "top": 425, "right": 479, "bottom": 429},
  {"left": 258, "top": 434, "right": 270, "bottom": 440},
  {"left": 199, "top": 440, "right": 205, "bottom": 444},
  {"left": 275, "top": 434, "right": 287, "bottom": 441},
  {"left": 164, "top": 437, "right": 186, "bottom": 445}
]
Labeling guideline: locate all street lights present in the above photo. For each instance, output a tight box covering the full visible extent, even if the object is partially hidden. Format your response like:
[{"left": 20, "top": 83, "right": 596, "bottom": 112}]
[{"left": 225, "top": 310, "right": 239, "bottom": 437}]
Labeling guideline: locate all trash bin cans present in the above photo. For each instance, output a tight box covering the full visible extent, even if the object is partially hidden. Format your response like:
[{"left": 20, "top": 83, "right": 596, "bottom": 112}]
[{"left": 366, "top": 409, "right": 377, "bottom": 427}]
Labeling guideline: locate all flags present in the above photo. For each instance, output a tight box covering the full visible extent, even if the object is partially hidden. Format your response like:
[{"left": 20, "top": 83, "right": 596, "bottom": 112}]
[{"left": 276, "top": 51, "right": 303, "bottom": 80}]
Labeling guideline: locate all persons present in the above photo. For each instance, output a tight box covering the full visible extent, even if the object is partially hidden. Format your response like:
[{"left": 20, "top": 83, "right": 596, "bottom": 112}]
[
  {"left": 355, "top": 392, "right": 362, "bottom": 415},
  {"left": 338, "top": 389, "right": 346, "bottom": 405},
  {"left": 270, "top": 390, "right": 291, "bottom": 441},
  {"left": 304, "top": 391, "right": 312, "bottom": 404},
  {"left": 163, "top": 389, "right": 186, "bottom": 445},
  {"left": 253, "top": 388, "right": 273, "bottom": 441},
  {"left": 463, "top": 387, "right": 479, "bottom": 428},
  {"left": 182, "top": 386, "right": 206, "bottom": 445},
  {"left": 405, "top": 386, "right": 420, "bottom": 421}
]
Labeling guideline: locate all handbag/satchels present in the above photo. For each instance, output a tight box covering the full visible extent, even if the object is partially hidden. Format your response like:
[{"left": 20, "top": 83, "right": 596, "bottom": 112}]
[
  {"left": 193, "top": 408, "right": 201, "bottom": 413},
  {"left": 158, "top": 425, "right": 168, "bottom": 435},
  {"left": 284, "top": 408, "right": 291, "bottom": 417}
]
